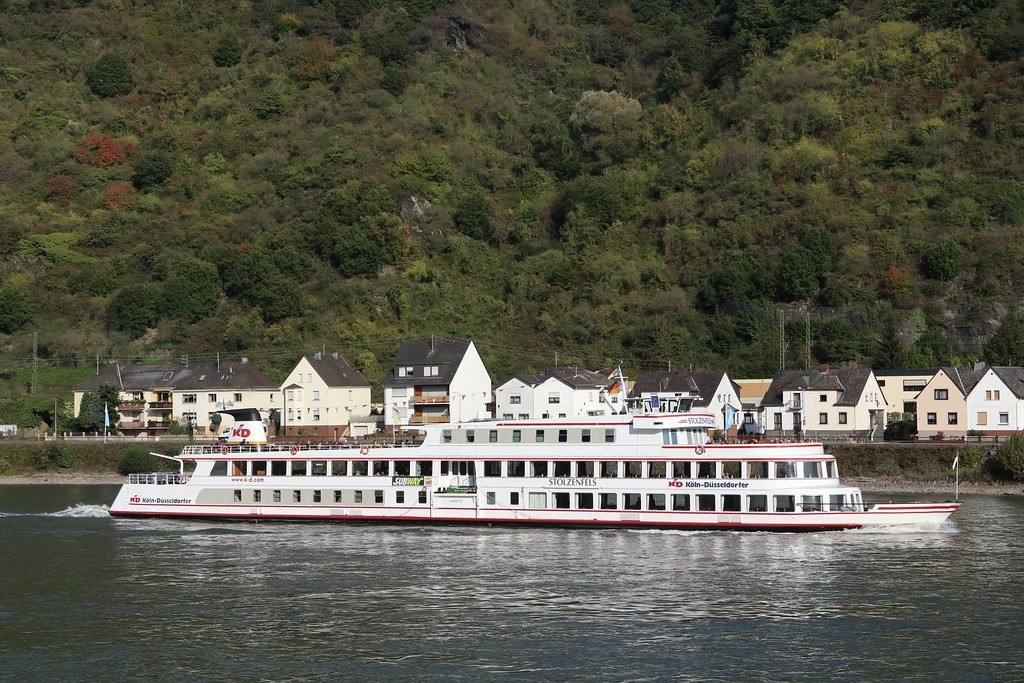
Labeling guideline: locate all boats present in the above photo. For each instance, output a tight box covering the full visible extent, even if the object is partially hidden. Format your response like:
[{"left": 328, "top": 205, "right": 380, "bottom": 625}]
[{"left": 109, "top": 366, "right": 961, "bottom": 533}]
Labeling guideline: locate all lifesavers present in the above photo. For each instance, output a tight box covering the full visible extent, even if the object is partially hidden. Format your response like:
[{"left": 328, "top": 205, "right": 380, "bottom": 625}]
[
  {"left": 222, "top": 447, "right": 229, "bottom": 453},
  {"left": 290, "top": 446, "right": 298, "bottom": 454},
  {"left": 360, "top": 445, "right": 369, "bottom": 455},
  {"left": 695, "top": 445, "right": 705, "bottom": 455}
]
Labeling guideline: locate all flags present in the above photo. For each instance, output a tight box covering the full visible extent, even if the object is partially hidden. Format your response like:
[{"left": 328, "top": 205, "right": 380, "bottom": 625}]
[
  {"left": 607, "top": 366, "right": 620, "bottom": 380},
  {"left": 607, "top": 381, "right": 620, "bottom": 396}
]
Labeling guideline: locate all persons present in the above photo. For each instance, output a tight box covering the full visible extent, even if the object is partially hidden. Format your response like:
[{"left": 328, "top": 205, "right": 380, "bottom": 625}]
[
  {"left": 376, "top": 470, "right": 384, "bottom": 476},
  {"left": 378, "top": 427, "right": 382, "bottom": 433},
  {"left": 705, "top": 434, "right": 816, "bottom": 444},
  {"left": 578, "top": 495, "right": 821, "bottom": 512},
  {"left": 207, "top": 439, "right": 414, "bottom": 454},
  {"left": 537, "top": 471, "right": 795, "bottom": 479},
  {"left": 356, "top": 470, "right": 365, "bottom": 476}
]
